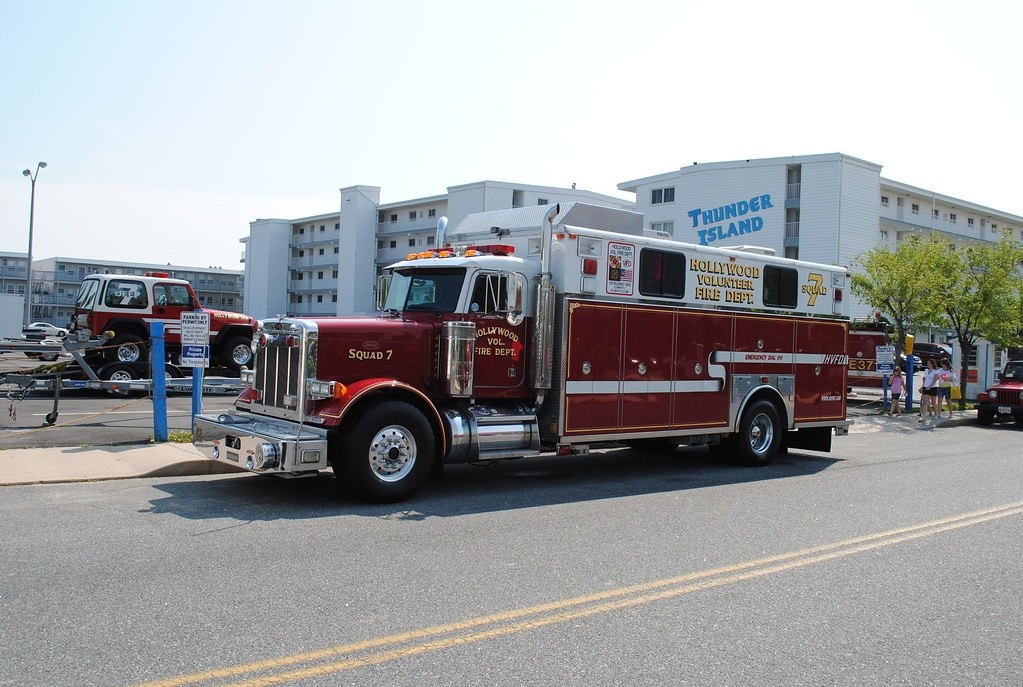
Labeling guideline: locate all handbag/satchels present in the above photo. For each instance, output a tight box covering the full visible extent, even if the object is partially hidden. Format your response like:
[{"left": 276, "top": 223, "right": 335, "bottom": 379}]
[{"left": 950, "top": 381, "right": 962, "bottom": 399}]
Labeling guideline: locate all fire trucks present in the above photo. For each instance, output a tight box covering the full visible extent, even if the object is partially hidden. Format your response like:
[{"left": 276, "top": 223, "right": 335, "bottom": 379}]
[{"left": 192, "top": 201, "right": 891, "bottom": 505}]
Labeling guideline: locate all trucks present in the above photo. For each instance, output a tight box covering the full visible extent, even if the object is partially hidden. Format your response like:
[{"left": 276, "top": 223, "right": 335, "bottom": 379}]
[{"left": 974, "top": 344, "right": 1023, "bottom": 426}]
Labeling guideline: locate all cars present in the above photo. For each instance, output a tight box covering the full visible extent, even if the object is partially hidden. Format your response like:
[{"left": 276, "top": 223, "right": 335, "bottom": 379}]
[
  {"left": 27, "top": 322, "right": 69, "bottom": 338},
  {"left": 892, "top": 342, "right": 922, "bottom": 375}
]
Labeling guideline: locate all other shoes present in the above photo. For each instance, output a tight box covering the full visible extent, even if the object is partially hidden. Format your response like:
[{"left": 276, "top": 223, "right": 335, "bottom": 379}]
[
  {"left": 895, "top": 413, "right": 900, "bottom": 416},
  {"left": 928, "top": 413, "right": 932, "bottom": 416},
  {"left": 917, "top": 413, "right": 921, "bottom": 416},
  {"left": 887, "top": 412, "right": 892, "bottom": 417},
  {"left": 933, "top": 415, "right": 938, "bottom": 418},
  {"left": 949, "top": 412, "right": 952, "bottom": 417},
  {"left": 918, "top": 418, "right": 925, "bottom": 422}
]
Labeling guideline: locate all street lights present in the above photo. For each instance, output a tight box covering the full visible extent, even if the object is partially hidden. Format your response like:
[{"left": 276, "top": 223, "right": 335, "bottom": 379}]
[{"left": 22, "top": 161, "right": 48, "bottom": 329}]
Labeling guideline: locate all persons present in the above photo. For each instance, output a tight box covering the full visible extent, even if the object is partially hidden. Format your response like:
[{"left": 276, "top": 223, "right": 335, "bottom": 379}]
[
  {"left": 875, "top": 310, "right": 891, "bottom": 324},
  {"left": 886, "top": 366, "right": 908, "bottom": 417},
  {"left": 916, "top": 358, "right": 957, "bottom": 422},
  {"left": 643, "top": 251, "right": 683, "bottom": 296}
]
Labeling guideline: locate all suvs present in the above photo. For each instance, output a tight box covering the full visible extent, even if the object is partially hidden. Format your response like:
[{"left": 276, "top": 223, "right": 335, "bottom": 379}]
[
  {"left": 911, "top": 342, "right": 953, "bottom": 368},
  {"left": 66, "top": 271, "right": 257, "bottom": 371}
]
